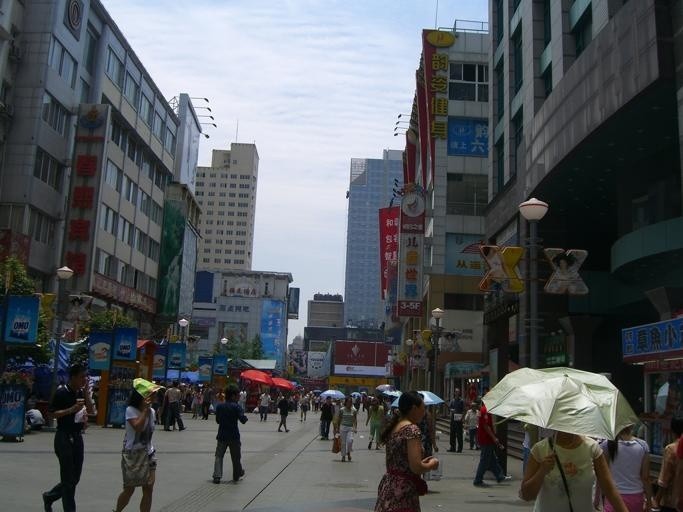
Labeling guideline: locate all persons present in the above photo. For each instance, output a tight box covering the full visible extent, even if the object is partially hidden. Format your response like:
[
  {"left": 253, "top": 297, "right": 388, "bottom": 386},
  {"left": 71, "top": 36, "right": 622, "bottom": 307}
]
[
  {"left": 320, "top": 394, "right": 400, "bottom": 462},
  {"left": 116, "top": 389, "right": 157, "bottom": 511},
  {"left": 27, "top": 408, "right": 45, "bottom": 431},
  {"left": 448, "top": 388, "right": 481, "bottom": 453},
  {"left": 150, "top": 378, "right": 225, "bottom": 432},
  {"left": 473, "top": 393, "right": 512, "bottom": 488},
  {"left": 212, "top": 384, "right": 249, "bottom": 484},
  {"left": 42, "top": 366, "right": 98, "bottom": 511},
  {"left": 238, "top": 386, "right": 321, "bottom": 433},
  {"left": 556, "top": 257, "right": 574, "bottom": 273},
  {"left": 374, "top": 390, "right": 439, "bottom": 510},
  {"left": 518, "top": 417, "right": 680, "bottom": 510}
]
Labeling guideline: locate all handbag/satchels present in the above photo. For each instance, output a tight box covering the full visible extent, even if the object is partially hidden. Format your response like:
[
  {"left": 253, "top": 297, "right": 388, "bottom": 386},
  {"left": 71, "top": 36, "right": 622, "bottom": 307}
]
[
  {"left": 120, "top": 448, "right": 151, "bottom": 490},
  {"left": 332, "top": 436, "right": 342, "bottom": 454},
  {"left": 54, "top": 437, "right": 73, "bottom": 468}
]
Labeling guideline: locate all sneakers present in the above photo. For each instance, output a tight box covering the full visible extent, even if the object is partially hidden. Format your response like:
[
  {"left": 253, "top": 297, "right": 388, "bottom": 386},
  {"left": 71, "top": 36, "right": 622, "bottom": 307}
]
[
  {"left": 497, "top": 475, "right": 513, "bottom": 484},
  {"left": 232, "top": 469, "right": 245, "bottom": 481},
  {"left": 474, "top": 479, "right": 491, "bottom": 488},
  {"left": 213, "top": 477, "right": 222, "bottom": 484}
]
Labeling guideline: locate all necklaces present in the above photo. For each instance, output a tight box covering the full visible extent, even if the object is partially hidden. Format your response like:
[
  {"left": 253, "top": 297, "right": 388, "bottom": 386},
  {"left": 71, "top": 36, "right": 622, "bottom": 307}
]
[{"left": 561, "top": 434, "right": 576, "bottom": 448}]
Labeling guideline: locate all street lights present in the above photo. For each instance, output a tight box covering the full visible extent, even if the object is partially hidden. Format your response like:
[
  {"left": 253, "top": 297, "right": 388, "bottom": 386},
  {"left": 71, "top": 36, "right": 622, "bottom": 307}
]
[
  {"left": 178, "top": 318, "right": 188, "bottom": 379},
  {"left": 220, "top": 337, "right": 228, "bottom": 353},
  {"left": 431, "top": 307, "right": 445, "bottom": 395},
  {"left": 517, "top": 195, "right": 548, "bottom": 367},
  {"left": 48, "top": 265, "right": 75, "bottom": 406}
]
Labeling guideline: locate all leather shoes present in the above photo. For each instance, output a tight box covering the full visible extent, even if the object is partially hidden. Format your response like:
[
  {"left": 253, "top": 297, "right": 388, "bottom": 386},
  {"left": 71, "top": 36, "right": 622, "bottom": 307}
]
[{"left": 42, "top": 492, "right": 55, "bottom": 512}]
